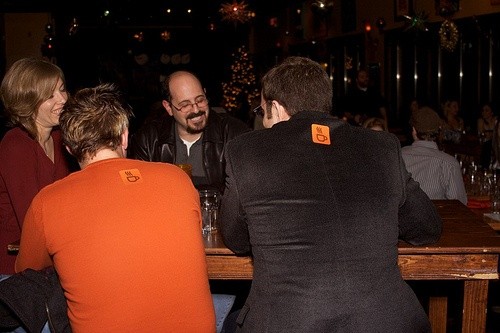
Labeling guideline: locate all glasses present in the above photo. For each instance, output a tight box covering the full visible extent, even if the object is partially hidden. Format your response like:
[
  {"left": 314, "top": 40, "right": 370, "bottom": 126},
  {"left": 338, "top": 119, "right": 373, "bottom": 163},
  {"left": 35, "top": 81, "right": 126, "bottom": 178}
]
[
  {"left": 253, "top": 100, "right": 272, "bottom": 119},
  {"left": 172, "top": 98, "right": 208, "bottom": 112}
]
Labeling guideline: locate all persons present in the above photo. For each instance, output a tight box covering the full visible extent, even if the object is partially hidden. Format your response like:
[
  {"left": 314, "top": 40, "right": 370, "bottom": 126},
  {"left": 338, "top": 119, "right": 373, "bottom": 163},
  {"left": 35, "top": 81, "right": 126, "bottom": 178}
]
[
  {"left": 218, "top": 56, "right": 442, "bottom": 333},
  {"left": 342, "top": 70, "right": 500, "bottom": 207},
  {"left": 0, "top": 58, "right": 78, "bottom": 333},
  {"left": 131, "top": 71, "right": 255, "bottom": 333},
  {"left": 14, "top": 84, "right": 217, "bottom": 333}
]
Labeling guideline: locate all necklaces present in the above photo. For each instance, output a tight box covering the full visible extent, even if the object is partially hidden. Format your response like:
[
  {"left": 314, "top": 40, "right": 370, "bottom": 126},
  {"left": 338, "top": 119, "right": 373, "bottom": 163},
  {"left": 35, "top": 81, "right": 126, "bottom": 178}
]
[{"left": 39, "top": 141, "right": 49, "bottom": 158}]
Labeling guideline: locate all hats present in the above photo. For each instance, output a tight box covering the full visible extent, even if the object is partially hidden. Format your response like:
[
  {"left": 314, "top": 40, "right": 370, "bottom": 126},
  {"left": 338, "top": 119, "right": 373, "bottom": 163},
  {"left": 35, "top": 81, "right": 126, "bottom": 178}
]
[{"left": 410, "top": 107, "right": 441, "bottom": 133}]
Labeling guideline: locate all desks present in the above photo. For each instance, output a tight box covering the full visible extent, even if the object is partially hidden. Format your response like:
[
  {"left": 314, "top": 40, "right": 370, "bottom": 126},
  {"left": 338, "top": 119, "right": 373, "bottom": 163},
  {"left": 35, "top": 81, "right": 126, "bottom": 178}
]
[{"left": 8, "top": 199, "right": 500, "bottom": 333}]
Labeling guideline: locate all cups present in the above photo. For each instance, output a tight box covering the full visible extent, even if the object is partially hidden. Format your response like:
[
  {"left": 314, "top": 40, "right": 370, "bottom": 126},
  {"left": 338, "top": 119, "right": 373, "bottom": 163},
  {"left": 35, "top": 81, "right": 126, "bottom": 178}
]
[
  {"left": 178, "top": 164, "right": 192, "bottom": 179},
  {"left": 200, "top": 190, "right": 221, "bottom": 231}
]
